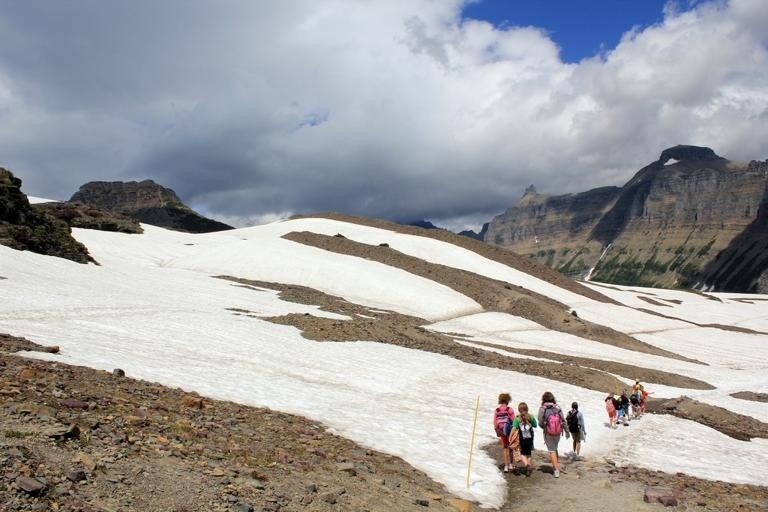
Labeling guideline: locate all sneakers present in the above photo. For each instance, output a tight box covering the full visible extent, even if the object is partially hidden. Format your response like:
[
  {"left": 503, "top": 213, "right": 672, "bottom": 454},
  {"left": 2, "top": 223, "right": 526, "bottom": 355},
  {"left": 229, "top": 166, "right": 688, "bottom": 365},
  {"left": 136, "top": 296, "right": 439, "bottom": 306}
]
[
  {"left": 573, "top": 453, "right": 583, "bottom": 461},
  {"left": 504, "top": 464, "right": 560, "bottom": 477}
]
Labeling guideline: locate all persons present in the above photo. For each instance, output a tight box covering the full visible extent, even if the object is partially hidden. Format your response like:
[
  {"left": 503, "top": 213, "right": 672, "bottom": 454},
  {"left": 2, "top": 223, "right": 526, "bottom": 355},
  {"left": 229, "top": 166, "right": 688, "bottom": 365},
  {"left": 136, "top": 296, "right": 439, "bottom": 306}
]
[
  {"left": 604, "top": 381, "right": 648, "bottom": 430},
  {"left": 511, "top": 402, "right": 537, "bottom": 477},
  {"left": 567, "top": 402, "right": 587, "bottom": 457},
  {"left": 494, "top": 393, "right": 515, "bottom": 472},
  {"left": 538, "top": 392, "right": 569, "bottom": 478}
]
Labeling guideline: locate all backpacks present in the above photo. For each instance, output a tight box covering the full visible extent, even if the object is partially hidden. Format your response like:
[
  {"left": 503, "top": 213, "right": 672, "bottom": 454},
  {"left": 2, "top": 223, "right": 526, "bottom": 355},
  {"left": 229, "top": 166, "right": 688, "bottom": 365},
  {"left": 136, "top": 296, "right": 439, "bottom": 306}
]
[
  {"left": 519, "top": 420, "right": 533, "bottom": 440},
  {"left": 494, "top": 407, "right": 512, "bottom": 437},
  {"left": 546, "top": 412, "right": 563, "bottom": 435},
  {"left": 566, "top": 411, "right": 579, "bottom": 433}
]
